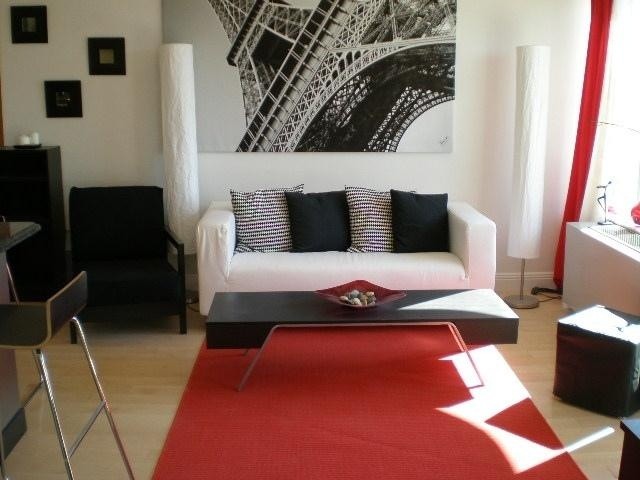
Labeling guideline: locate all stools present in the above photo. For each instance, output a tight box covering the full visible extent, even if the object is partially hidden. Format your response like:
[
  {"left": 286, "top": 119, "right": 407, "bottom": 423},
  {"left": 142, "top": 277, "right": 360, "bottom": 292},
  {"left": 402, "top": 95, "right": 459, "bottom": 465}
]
[
  {"left": 0, "top": 269, "right": 134, "bottom": 480},
  {"left": 552, "top": 304, "right": 640, "bottom": 417}
]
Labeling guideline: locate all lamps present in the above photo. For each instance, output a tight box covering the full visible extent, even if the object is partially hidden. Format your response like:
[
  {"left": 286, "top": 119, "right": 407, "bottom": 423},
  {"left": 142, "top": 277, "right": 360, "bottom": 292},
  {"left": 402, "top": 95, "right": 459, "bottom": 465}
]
[
  {"left": 158, "top": 44, "right": 201, "bottom": 303},
  {"left": 504, "top": 44, "right": 551, "bottom": 309}
]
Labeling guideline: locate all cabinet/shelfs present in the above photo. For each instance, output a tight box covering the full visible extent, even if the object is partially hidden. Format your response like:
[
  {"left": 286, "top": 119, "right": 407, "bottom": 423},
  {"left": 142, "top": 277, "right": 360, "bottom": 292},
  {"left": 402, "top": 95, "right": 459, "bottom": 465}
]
[{"left": 1, "top": 145, "right": 63, "bottom": 277}]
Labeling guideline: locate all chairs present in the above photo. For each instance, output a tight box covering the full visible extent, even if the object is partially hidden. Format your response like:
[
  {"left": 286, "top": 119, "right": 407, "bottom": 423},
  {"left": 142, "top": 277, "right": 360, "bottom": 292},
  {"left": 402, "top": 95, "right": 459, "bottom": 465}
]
[{"left": 57, "top": 186, "right": 187, "bottom": 344}]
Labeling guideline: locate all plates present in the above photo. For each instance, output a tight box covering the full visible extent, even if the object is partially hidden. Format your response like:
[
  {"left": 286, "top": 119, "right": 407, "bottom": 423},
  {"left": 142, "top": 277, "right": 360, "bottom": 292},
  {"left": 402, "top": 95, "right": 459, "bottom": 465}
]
[
  {"left": 14, "top": 144, "right": 41, "bottom": 148},
  {"left": 314, "top": 279, "right": 407, "bottom": 311}
]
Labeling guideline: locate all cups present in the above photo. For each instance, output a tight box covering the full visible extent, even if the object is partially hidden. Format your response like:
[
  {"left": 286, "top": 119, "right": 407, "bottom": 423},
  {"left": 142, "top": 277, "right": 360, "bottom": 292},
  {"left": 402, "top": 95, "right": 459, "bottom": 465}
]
[{"left": 18, "top": 132, "right": 39, "bottom": 145}]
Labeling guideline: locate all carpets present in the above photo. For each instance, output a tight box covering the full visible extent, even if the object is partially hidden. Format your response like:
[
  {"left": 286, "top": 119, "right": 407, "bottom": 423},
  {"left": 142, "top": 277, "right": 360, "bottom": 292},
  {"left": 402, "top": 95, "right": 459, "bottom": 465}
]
[{"left": 150, "top": 326, "right": 588, "bottom": 480}]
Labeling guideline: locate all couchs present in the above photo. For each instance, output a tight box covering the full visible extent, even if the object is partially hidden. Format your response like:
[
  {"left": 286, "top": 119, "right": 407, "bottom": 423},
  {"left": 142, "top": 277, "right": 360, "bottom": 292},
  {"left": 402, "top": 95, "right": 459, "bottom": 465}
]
[{"left": 196, "top": 200, "right": 497, "bottom": 316}]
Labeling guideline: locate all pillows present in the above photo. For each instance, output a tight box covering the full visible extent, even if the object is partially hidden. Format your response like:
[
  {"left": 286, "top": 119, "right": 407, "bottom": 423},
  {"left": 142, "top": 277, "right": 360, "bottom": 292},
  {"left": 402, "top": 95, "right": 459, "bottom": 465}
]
[
  {"left": 284, "top": 191, "right": 350, "bottom": 252},
  {"left": 390, "top": 189, "right": 449, "bottom": 253},
  {"left": 345, "top": 186, "right": 416, "bottom": 253},
  {"left": 230, "top": 184, "right": 304, "bottom": 255}
]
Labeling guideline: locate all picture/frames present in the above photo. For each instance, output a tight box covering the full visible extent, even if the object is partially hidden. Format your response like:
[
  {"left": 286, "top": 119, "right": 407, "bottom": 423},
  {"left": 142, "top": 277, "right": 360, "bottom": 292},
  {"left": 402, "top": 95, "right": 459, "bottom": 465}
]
[
  {"left": 10, "top": 5, "right": 48, "bottom": 44},
  {"left": 43, "top": 80, "right": 83, "bottom": 118},
  {"left": 87, "top": 37, "right": 126, "bottom": 76}
]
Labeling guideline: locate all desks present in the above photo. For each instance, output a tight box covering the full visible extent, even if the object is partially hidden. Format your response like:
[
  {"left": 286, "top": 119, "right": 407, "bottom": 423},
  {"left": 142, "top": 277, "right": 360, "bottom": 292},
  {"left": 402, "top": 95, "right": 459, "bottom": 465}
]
[
  {"left": 205, "top": 289, "right": 520, "bottom": 392},
  {"left": 618, "top": 416, "right": 639, "bottom": 480},
  {"left": 0, "top": 221, "right": 43, "bottom": 462}
]
